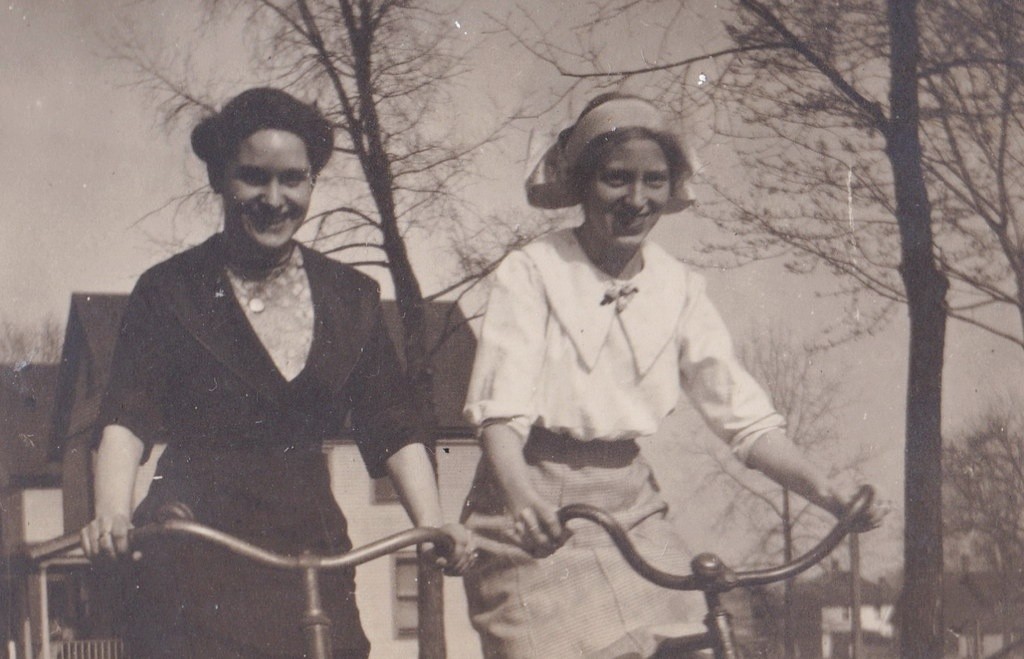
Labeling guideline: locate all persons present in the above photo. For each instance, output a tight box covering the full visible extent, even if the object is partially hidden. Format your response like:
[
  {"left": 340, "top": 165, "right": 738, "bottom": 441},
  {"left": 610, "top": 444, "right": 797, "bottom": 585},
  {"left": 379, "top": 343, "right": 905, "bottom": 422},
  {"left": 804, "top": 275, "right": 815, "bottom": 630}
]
[
  {"left": 81, "top": 88, "right": 480, "bottom": 659},
  {"left": 463, "top": 94, "right": 892, "bottom": 659}
]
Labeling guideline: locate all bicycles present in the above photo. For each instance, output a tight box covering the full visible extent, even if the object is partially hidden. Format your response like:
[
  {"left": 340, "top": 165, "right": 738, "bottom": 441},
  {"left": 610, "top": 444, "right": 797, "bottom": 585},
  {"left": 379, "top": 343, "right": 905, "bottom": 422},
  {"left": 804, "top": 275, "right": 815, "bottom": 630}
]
[
  {"left": 554, "top": 485, "right": 873, "bottom": 658},
  {"left": 129, "top": 517, "right": 456, "bottom": 658}
]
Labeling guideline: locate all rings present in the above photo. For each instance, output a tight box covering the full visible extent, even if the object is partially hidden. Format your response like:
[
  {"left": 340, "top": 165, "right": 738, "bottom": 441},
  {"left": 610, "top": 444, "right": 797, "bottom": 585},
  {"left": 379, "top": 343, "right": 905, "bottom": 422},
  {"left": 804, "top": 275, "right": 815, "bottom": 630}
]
[
  {"left": 463, "top": 548, "right": 471, "bottom": 557},
  {"left": 529, "top": 524, "right": 539, "bottom": 533},
  {"left": 99, "top": 531, "right": 110, "bottom": 537}
]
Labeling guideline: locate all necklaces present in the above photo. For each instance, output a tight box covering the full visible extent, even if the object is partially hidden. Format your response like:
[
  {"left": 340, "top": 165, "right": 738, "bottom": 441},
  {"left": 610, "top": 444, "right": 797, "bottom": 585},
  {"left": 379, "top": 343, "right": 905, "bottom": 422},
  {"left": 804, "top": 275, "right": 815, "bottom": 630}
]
[{"left": 226, "top": 244, "right": 297, "bottom": 314}]
